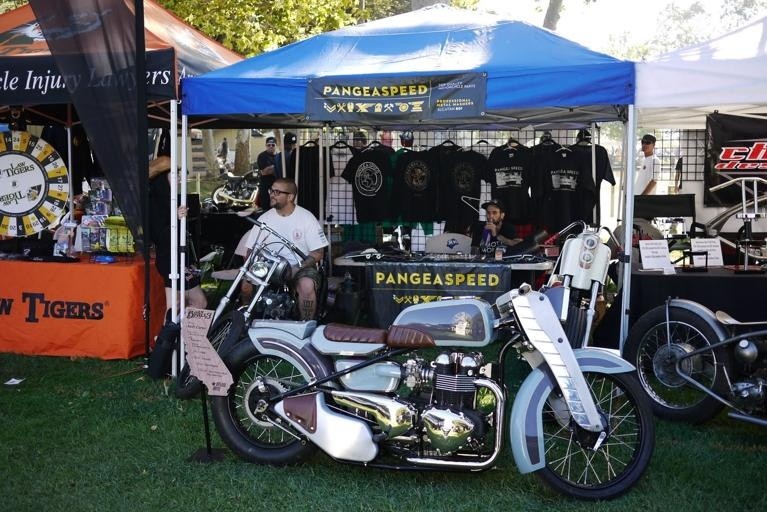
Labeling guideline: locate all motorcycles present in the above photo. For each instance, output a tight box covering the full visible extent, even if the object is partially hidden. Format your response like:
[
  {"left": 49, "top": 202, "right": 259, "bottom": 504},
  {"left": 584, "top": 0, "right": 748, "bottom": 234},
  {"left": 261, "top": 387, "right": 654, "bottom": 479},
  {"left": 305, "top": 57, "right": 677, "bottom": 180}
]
[
  {"left": 621, "top": 296, "right": 767, "bottom": 428},
  {"left": 206, "top": 170, "right": 260, "bottom": 209},
  {"left": 148, "top": 217, "right": 307, "bottom": 398},
  {"left": 207, "top": 223, "right": 654, "bottom": 500}
]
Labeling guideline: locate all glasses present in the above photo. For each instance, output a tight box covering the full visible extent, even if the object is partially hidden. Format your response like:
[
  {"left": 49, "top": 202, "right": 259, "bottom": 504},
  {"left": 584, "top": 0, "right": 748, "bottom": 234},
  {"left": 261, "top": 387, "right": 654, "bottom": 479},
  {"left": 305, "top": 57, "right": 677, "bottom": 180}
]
[
  {"left": 268, "top": 187, "right": 294, "bottom": 196},
  {"left": 266, "top": 143, "right": 276, "bottom": 147},
  {"left": 641, "top": 141, "right": 653, "bottom": 145}
]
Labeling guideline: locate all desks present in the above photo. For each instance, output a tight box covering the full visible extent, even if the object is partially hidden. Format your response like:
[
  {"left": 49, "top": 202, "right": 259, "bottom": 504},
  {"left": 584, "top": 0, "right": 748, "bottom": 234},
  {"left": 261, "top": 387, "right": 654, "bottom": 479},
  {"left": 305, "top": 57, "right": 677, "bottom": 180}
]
[
  {"left": 605, "top": 264, "right": 766, "bottom": 345},
  {"left": 0, "top": 254, "right": 166, "bottom": 359},
  {"left": 334, "top": 248, "right": 553, "bottom": 322}
]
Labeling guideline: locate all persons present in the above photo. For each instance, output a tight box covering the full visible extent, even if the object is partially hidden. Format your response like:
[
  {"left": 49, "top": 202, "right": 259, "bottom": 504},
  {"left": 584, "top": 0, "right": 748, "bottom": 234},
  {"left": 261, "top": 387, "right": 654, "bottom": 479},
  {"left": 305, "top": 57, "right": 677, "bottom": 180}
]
[
  {"left": 674, "top": 157, "right": 682, "bottom": 193},
  {"left": 637, "top": 135, "right": 660, "bottom": 196},
  {"left": 145, "top": 156, "right": 208, "bottom": 328},
  {"left": 241, "top": 176, "right": 329, "bottom": 321},
  {"left": 473, "top": 200, "right": 522, "bottom": 254},
  {"left": 258, "top": 133, "right": 298, "bottom": 177},
  {"left": 222, "top": 137, "right": 229, "bottom": 160}
]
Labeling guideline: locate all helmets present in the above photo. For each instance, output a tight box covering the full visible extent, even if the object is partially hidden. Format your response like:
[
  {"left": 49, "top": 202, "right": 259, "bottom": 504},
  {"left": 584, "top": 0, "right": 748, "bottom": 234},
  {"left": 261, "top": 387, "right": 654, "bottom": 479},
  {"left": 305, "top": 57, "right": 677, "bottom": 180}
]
[{"left": 149, "top": 156, "right": 182, "bottom": 179}]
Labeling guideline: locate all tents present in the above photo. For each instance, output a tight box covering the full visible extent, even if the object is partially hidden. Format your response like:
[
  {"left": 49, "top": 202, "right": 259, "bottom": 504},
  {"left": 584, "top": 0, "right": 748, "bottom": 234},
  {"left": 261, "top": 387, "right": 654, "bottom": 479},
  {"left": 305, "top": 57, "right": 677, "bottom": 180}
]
[
  {"left": 1, "top": 2, "right": 250, "bottom": 256},
  {"left": 627, "top": 16, "right": 763, "bottom": 382},
  {"left": 182, "top": 4, "right": 636, "bottom": 377}
]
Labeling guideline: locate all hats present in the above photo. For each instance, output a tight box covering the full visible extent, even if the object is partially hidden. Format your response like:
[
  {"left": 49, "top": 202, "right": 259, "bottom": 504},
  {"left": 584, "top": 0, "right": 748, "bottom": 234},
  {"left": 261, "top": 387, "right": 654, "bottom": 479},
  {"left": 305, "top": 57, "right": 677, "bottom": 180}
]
[
  {"left": 481, "top": 200, "right": 509, "bottom": 214},
  {"left": 642, "top": 133, "right": 658, "bottom": 144},
  {"left": 265, "top": 136, "right": 278, "bottom": 145},
  {"left": 284, "top": 131, "right": 297, "bottom": 144}
]
